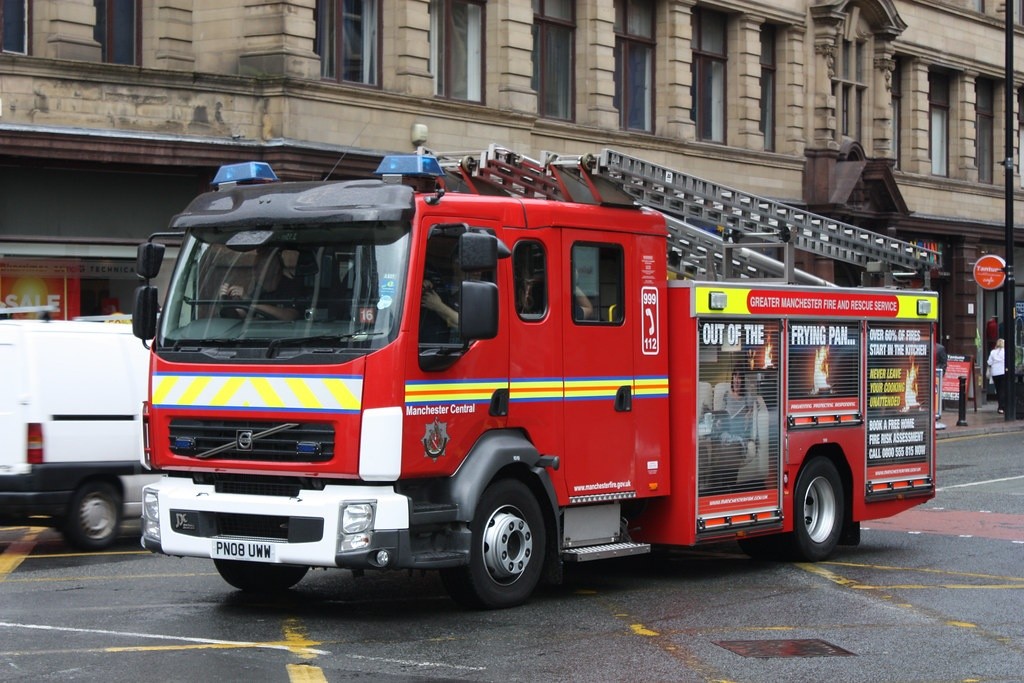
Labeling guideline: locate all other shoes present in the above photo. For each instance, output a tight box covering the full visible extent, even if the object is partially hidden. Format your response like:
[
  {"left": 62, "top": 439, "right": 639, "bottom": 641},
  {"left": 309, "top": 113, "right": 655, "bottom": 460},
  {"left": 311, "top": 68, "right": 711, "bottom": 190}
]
[{"left": 998, "top": 409, "right": 1006, "bottom": 414}]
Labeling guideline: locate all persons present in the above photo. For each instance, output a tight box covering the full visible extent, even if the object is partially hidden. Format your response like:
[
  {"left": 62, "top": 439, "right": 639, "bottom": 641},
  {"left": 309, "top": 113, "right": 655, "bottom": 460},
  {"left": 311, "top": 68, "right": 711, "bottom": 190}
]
[
  {"left": 421, "top": 245, "right": 482, "bottom": 344},
  {"left": 220, "top": 250, "right": 306, "bottom": 320},
  {"left": 987, "top": 338, "right": 1005, "bottom": 413},
  {"left": 104, "top": 305, "right": 132, "bottom": 325},
  {"left": 936, "top": 343, "right": 948, "bottom": 377},
  {"left": 576, "top": 270, "right": 594, "bottom": 320},
  {"left": 715, "top": 362, "right": 753, "bottom": 479}
]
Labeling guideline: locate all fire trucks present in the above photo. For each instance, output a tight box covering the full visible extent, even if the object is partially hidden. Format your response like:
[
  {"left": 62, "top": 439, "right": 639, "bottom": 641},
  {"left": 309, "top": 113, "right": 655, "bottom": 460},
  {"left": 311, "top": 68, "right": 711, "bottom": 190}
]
[{"left": 131, "top": 144, "right": 941, "bottom": 610}]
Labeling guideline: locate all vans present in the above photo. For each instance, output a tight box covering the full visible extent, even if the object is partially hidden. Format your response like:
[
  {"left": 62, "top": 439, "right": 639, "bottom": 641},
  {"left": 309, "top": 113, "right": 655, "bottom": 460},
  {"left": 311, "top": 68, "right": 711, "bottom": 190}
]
[{"left": 0, "top": 303, "right": 152, "bottom": 553}]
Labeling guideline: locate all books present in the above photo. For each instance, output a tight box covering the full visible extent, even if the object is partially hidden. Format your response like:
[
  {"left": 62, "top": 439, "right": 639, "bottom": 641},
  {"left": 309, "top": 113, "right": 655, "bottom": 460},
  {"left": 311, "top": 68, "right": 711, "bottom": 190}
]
[{"left": 712, "top": 405, "right": 749, "bottom": 423}]
